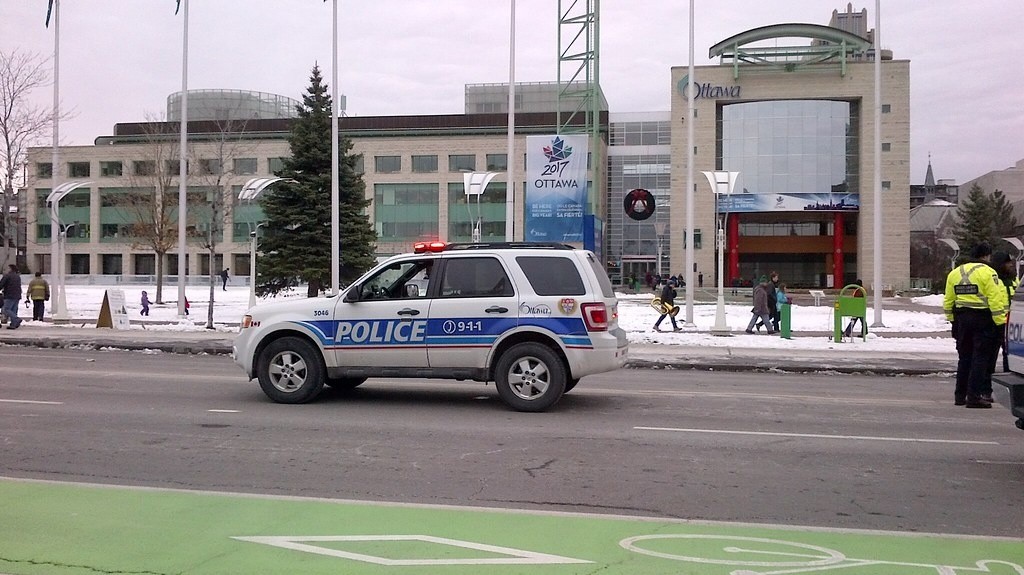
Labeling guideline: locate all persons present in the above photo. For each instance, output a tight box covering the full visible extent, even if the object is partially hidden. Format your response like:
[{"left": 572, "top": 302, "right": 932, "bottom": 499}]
[
  {"left": 221, "top": 268, "right": 231, "bottom": 291},
  {"left": 185, "top": 296, "right": 189, "bottom": 315},
  {"left": 944, "top": 242, "right": 1020, "bottom": 408},
  {"left": 745, "top": 271, "right": 793, "bottom": 334},
  {"left": 653, "top": 280, "right": 682, "bottom": 332},
  {"left": 0, "top": 264, "right": 22, "bottom": 330},
  {"left": 732, "top": 276, "right": 738, "bottom": 296},
  {"left": 698, "top": 272, "right": 703, "bottom": 287},
  {"left": 140, "top": 291, "right": 152, "bottom": 316},
  {"left": 841, "top": 279, "right": 867, "bottom": 338},
  {"left": 645, "top": 271, "right": 686, "bottom": 290},
  {"left": 27, "top": 271, "right": 50, "bottom": 322}
]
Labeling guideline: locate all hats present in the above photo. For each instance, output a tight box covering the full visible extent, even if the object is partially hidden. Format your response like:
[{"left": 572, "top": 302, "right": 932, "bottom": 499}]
[
  {"left": 666, "top": 280, "right": 675, "bottom": 286},
  {"left": 760, "top": 274, "right": 767, "bottom": 282},
  {"left": 777, "top": 282, "right": 786, "bottom": 289}
]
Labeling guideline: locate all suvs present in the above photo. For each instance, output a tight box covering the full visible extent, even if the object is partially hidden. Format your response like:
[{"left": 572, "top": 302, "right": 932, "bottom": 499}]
[{"left": 233, "top": 241, "right": 630, "bottom": 413}]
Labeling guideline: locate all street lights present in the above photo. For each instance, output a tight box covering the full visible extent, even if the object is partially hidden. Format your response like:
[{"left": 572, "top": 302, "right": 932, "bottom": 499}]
[
  {"left": 464, "top": 171, "right": 503, "bottom": 244},
  {"left": 46, "top": 179, "right": 95, "bottom": 320},
  {"left": 238, "top": 177, "right": 293, "bottom": 308},
  {"left": 936, "top": 238, "right": 961, "bottom": 270},
  {"left": 654, "top": 223, "right": 665, "bottom": 287},
  {"left": 1000, "top": 237, "right": 1024, "bottom": 281},
  {"left": 700, "top": 170, "right": 740, "bottom": 331}
]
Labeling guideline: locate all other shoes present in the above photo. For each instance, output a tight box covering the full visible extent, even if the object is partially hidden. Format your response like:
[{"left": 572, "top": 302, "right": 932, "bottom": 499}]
[
  {"left": 768, "top": 331, "right": 777, "bottom": 334},
  {"left": 756, "top": 323, "right": 760, "bottom": 331},
  {"left": 842, "top": 331, "right": 849, "bottom": 338},
  {"left": 857, "top": 333, "right": 868, "bottom": 338},
  {"left": 745, "top": 329, "right": 755, "bottom": 334},
  {"left": 773, "top": 330, "right": 781, "bottom": 332}
]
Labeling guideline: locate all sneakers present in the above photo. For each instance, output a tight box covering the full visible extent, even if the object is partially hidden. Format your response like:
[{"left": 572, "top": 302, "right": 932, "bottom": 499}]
[
  {"left": 954, "top": 398, "right": 966, "bottom": 405},
  {"left": 14, "top": 317, "right": 22, "bottom": 328},
  {"left": 982, "top": 394, "right": 994, "bottom": 403},
  {"left": 966, "top": 395, "right": 992, "bottom": 408},
  {"left": 6, "top": 324, "right": 15, "bottom": 329},
  {"left": 673, "top": 327, "right": 682, "bottom": 332},
  {"left": 652, "top": 325, "right": 661, "bottom": 332}
]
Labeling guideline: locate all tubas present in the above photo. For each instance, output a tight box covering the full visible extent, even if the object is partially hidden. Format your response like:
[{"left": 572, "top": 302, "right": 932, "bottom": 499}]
[{"left": 651, "top": 297, "right": 680, "bottom": 317}]
